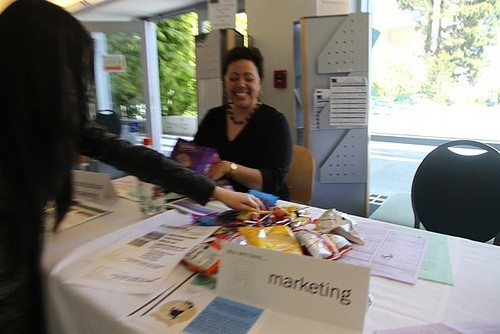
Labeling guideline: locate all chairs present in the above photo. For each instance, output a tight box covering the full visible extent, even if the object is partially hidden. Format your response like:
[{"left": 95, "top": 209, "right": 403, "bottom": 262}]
[
  {"left": 94, "top": 109, "right": 121, "bottom": 137},
  {"left": 285, "top": 144, "right": 316, "bottom": 206},
  {"left": 410, "top": 139, "right": 500, "bottom": 245}
]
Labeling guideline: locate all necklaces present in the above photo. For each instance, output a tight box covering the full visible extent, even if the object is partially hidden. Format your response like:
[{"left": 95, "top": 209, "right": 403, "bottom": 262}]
[{"left": 228, "top": 104, "right": 258, "bottom": 126}]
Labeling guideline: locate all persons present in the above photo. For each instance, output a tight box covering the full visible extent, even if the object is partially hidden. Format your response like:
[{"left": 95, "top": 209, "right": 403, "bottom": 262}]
[
  {"left": 0, "top": 0, "right": 267, "bottom": 334},
  {"left": 192, "top": 45, "right": 290, "bottom": 203}
]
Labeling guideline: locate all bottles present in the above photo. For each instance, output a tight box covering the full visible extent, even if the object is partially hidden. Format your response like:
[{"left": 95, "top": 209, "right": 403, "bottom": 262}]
[{"left": 137, "top": 138, "right": 165, "bottom": 216}]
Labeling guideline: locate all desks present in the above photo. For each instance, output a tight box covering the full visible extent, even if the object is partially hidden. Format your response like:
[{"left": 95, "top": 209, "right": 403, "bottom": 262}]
[{"left": 40, "top": 175, "right": 500, "bottom": 334}]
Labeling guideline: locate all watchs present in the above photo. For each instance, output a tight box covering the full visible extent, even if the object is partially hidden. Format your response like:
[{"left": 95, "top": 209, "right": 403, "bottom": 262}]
[{"left": 224, "top": 161, "right": 238, "bottom": 177}]
